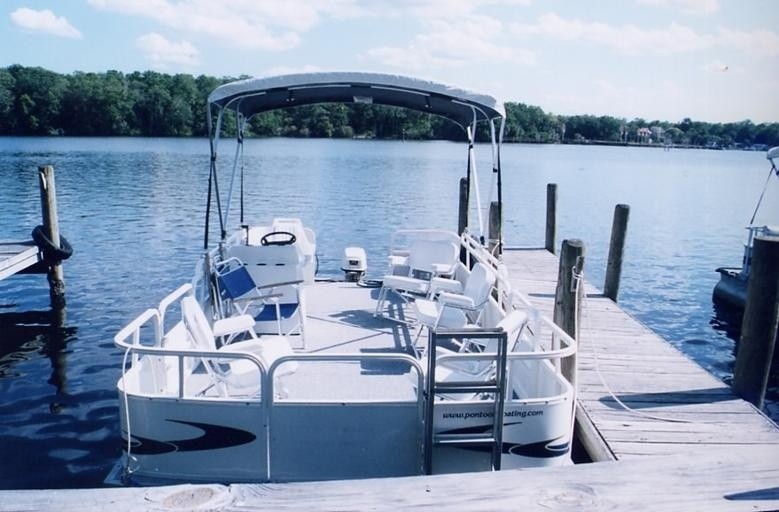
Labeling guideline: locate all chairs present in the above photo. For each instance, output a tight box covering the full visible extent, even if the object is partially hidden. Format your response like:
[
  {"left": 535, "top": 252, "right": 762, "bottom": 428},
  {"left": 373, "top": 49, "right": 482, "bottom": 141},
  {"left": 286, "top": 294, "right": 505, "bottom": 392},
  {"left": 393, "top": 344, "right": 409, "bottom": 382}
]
[
  {"left": 373, "top": 241, "right": 528, "bottom": 404},
  {"left": 179, "top": 256, "right": 306, "bottom": 399}
]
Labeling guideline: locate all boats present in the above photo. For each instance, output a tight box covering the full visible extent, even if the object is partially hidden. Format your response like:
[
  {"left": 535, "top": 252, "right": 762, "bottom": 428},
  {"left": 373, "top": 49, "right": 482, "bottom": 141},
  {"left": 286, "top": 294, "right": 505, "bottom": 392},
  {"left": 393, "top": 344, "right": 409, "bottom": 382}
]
[
  {"left": 712, "top": 146, "right": 779, "bottom": 307},
  {"left": 102, "top": 72, "right": 577, "bottom": 487}
]
